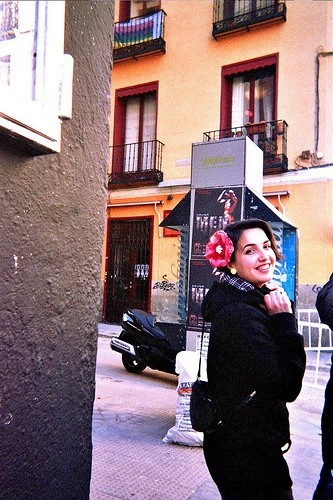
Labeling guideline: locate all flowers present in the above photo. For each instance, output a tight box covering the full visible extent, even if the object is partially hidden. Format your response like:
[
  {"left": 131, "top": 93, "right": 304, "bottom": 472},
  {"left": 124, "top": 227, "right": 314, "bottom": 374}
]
[{"left": 206, "top": 230, "right": 235, "bottom": 268}]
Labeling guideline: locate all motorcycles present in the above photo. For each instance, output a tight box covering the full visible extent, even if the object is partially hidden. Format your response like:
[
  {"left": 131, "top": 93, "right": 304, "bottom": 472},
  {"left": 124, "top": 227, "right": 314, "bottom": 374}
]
[{"left": 109, "top": 307, "right": 182, "bottom": 378}]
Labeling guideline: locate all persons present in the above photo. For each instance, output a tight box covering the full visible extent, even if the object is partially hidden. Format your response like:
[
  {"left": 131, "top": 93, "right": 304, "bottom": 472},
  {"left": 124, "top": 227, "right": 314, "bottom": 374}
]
[
  {"left": 201, "top": 218, "right": 308, "bottom": 500},
  {"left": 311, "top": 271, "right": 333, "bottom": 500}
]
